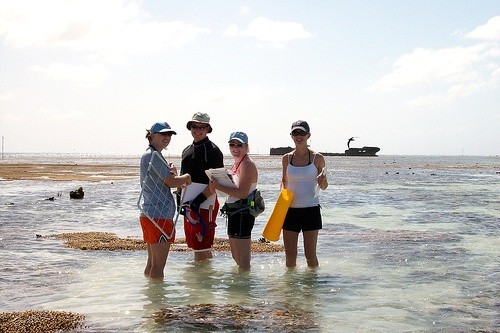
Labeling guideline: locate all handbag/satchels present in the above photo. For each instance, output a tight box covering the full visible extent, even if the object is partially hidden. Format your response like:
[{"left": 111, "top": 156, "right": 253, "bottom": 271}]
[{"left": 247, "top": 190, "right": 265, "bottom": 216}]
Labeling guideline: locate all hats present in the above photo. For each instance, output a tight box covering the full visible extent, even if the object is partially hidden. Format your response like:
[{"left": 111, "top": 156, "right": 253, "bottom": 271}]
[
  {"left": 228, "top": 132, "right": 248, "bottom": 144},
  {"left": 187, "top": 112, "right": 212, "bottom": 133},
  {"left": 151, "top": 122, "right": 177, "bottom": 135},
  {"left": 290, "top": 120, "right": 310, "bottom": 132}
]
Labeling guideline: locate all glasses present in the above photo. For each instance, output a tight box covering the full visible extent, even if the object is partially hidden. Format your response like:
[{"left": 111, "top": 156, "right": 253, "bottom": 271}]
[
  {"left": 156, "top": 133, "right": 172, "bottom": 137},
  {"left": 230, "top": 144, "right": 245, "bottom": 147},
  {"left": 292, "top": 131, "right": 308, "bottom": 137},
  {"left": 190, "top": 125, "right": 207, "bottom": 130}
]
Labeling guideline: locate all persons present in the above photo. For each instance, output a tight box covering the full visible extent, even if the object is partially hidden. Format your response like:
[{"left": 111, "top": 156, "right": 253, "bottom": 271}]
[
  {"left": 173, "top": 112, "right": 224, "bottom": 260},
  {"left": 282, "top": 120, "right": 329, "bottom": 266},
  {"left": 139, "top": 122, "right": 191, "bottom": 278},
  {"left": 209, "top": 131, "right": 258, "bottom": 269}
]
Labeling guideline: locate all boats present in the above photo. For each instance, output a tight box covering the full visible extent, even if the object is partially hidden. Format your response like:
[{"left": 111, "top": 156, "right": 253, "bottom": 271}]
[{"left": 270, "top": 146, "right": 380, "bottom": 156}]
